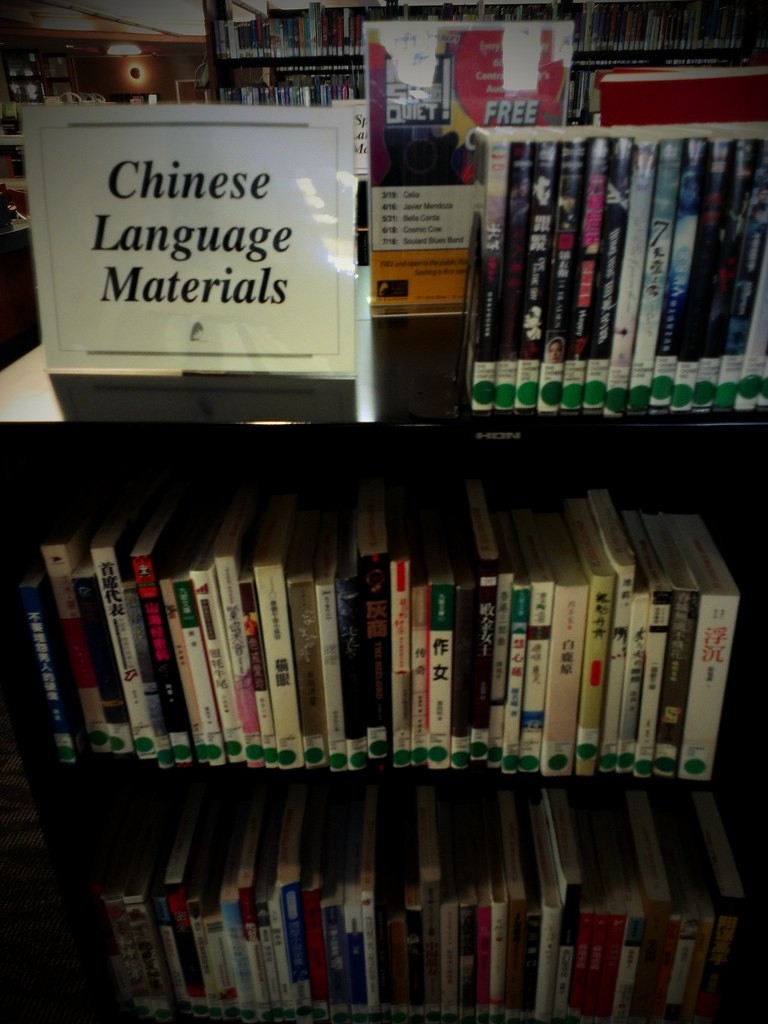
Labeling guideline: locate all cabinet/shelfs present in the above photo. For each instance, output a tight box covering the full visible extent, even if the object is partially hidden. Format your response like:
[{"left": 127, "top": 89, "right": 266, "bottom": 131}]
[{"left": 0, "top": 0, "right": 768, "bottom": 1024}]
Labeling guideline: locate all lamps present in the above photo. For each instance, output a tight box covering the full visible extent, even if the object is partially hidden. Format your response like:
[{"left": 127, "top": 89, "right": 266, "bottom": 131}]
[{"left": 107, "top": 44, "right": 142, "bottom": 57}]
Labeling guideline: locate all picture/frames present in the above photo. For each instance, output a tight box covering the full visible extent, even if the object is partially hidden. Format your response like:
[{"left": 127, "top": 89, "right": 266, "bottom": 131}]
[{"left": 19, "top": 104, "right": 357, "bottom": 377}]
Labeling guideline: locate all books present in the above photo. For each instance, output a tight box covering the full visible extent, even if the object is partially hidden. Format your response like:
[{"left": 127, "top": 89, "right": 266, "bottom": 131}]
[
  {"left": 213, "top": 1, "right": 768, "bottom": 60},
  {"left": 459, "top": 124, "right": 768, "bottom": 417},
  {"left": 89, "top": 785, "right": 747, "bottom": 1024},
  {"left": 19, "top": 476, "right": 743, "bottom": 779},
  {"left": 217, "top": 70, "right": 588, "bottom": 125}
]
